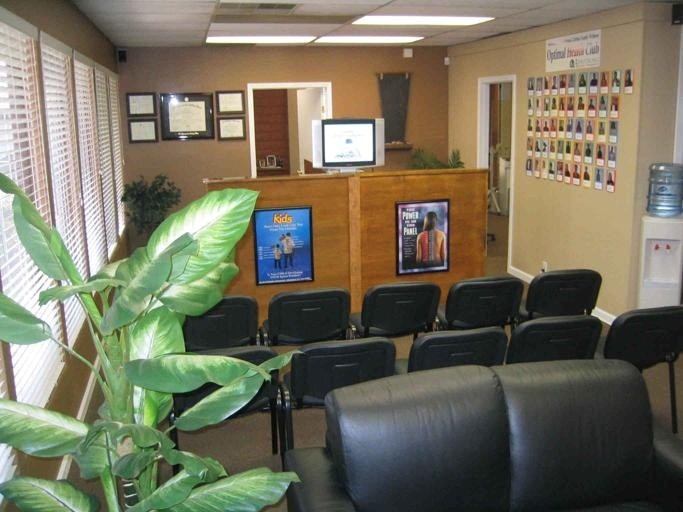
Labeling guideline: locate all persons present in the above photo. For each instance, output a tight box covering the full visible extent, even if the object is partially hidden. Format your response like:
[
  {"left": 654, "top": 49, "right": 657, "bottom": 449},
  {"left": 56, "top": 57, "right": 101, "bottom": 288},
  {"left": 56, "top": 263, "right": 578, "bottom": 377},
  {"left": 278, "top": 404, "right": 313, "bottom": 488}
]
[
  {"left": 415, "top": 211, "right": 445, "bottom": 267},
  {"left": 524, "top": 71, "right": 633, "bottom": 185},
  {"left": 273, "top": 232, "right": 296, "bottom": 269}
]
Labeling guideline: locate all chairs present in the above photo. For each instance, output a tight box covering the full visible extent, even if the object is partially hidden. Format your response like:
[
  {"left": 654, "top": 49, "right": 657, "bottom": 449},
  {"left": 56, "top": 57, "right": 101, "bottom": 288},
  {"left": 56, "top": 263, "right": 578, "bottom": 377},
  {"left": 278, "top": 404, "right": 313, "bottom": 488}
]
[
  {"left": 283, "top": 360, "right": 683, "bottom": 511},
  {"left": 170, "top": 271, "right": 683, "bottom": 474}
]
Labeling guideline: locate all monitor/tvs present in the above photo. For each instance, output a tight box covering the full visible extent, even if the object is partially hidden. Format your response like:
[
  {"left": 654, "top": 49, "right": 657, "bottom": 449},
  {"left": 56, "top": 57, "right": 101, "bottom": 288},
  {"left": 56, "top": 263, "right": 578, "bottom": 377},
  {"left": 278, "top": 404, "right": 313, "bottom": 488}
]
[{"left": 312, "top": 118, "right": 385, "bottom": 173}]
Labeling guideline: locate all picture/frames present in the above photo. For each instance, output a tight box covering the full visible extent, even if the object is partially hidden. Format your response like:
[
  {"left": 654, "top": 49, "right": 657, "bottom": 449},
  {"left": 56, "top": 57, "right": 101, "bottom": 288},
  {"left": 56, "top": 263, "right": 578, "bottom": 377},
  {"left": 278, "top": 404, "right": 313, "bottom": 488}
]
[
  {"left": 126, "top": 92, "right": 157, "bottom": 116},
  {"left": 215, "top": 91, "right": 245, "bottom": 115},
  {"left": 252, "top": 205, "right": 314, "bottom": 286},
  {"left": 395, "top": 198, "right": 449, "bottom": 275},
  {"left": 260, "top": 155, "right": 276, "bottom": 168},
  {"left": 128, "top": 119, "right": 158, "bottom": 143},
  {"left": 216, "top": 117, "right": 246, "bottom": 141},
  {"left": 160, "top": 92, "right": 215, "bottom": 140}
]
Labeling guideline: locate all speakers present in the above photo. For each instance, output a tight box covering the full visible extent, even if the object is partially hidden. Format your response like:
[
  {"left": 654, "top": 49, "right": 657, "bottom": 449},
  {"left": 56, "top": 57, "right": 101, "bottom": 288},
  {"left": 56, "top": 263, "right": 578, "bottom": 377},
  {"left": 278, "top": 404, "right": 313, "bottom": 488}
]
[
  {"left": 118, "top": 50, "right": 127, "bottom": 63},
  {"left": 671, "top": 3, "right": 683, "bottom": 24}
]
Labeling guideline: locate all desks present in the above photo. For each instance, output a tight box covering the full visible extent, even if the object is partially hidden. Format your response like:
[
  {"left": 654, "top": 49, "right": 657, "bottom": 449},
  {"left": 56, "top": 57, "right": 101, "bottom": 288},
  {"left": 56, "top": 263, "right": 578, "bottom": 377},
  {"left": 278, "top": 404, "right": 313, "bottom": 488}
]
[{"left": 258, "top": 167, "right": 287, "bottom": 177}]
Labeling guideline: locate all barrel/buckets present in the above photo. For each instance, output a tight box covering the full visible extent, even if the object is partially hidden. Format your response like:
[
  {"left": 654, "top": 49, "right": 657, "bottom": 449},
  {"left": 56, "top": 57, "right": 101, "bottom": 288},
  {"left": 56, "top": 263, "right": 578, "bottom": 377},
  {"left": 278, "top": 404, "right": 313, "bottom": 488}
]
[{"left": 646, "top": 162, "right": 683, "bottom": 219}]
